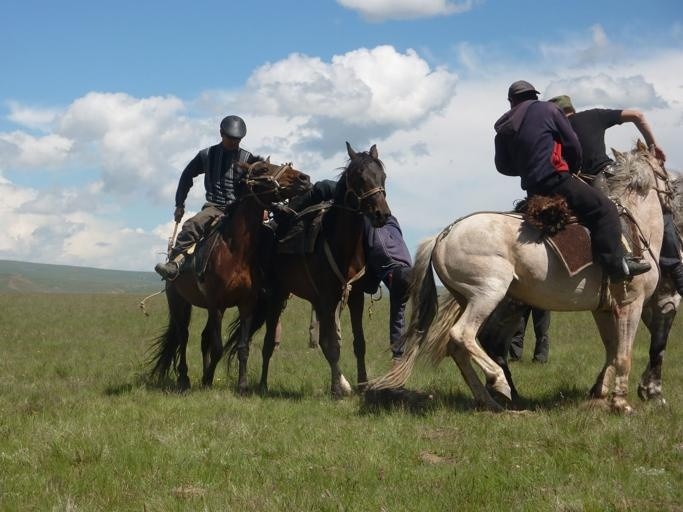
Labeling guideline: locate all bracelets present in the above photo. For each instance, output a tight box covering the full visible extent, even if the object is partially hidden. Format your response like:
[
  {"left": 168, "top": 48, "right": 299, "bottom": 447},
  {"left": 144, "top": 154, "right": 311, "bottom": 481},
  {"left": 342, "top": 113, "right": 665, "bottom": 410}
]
[{"left": 648, "top": 142, "right": 658, "bottom": 150}]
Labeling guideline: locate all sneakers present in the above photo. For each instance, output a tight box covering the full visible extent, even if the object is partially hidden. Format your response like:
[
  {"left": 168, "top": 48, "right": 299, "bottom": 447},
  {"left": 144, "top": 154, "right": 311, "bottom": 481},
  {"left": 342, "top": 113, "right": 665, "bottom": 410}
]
[
  {"left": 156, "top": 260, "right": 180, "bottom": 278},
  {"left": 609, "top": 261, "right": 650, "bottom": 284}
]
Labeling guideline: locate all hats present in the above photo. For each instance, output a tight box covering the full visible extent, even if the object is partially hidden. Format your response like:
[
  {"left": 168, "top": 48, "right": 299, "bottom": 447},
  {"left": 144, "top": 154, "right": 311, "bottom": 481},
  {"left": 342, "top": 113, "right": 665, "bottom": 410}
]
[
  {"left": 548, "top": 95, "right": 572, "bottom": 113},
  {"left": 222, "top": 115, "right": 247, "bottom": 139},
  {"left": 509, "top": 80, "right": 540, "bottom": 98}
]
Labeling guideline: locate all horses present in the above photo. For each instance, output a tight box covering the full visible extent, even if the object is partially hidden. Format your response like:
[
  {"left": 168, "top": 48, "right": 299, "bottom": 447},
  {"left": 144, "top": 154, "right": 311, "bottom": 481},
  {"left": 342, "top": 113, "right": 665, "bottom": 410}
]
[
  {"left": 220, "top": 140, "right": 391, "bottom": 397},
  {"left": 139, "top": 156, "right": 311, "bottom": 398},
  {"left": 353, "top": 141, "right": 683, "bottom": 418}
]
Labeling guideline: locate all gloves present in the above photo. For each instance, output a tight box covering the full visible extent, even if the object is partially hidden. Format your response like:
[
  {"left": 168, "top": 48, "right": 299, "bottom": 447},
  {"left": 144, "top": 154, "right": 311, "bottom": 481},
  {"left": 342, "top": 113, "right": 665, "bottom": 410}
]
[{"left": 174, "top": 207, "right": 184, "bottom": 222}]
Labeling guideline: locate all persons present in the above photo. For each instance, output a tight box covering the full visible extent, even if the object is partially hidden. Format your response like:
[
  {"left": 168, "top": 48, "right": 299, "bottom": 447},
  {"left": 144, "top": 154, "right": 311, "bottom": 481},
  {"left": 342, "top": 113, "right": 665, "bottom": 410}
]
[
  {"left": 274, "top": 179, "right": 414, "bottom": 372},
  {"left": 491, "top": 80, "right": 654, "bottom": 283},
  {"left": 549, "top": 94, "right": 668, "bottom": 262},
  {"left": 153, "top": 115, "right": 274, "bottom": 286},
  {"left": 507, "top": 304, "right": 553, "bottom": 366}
]
